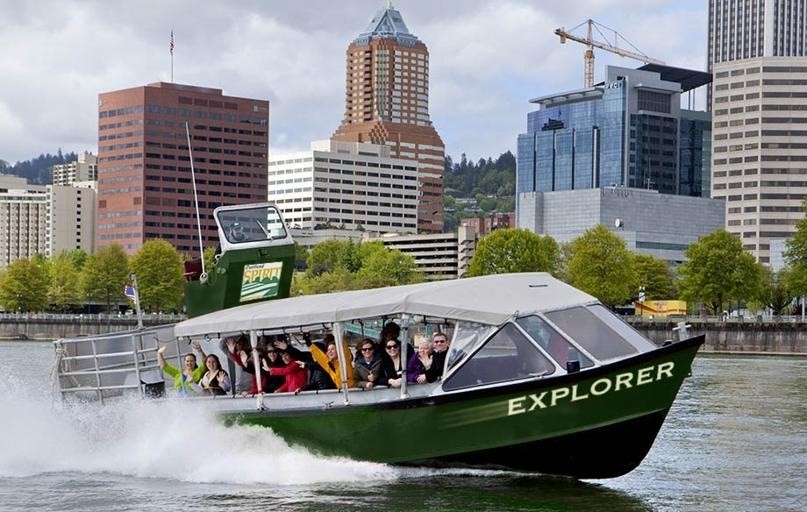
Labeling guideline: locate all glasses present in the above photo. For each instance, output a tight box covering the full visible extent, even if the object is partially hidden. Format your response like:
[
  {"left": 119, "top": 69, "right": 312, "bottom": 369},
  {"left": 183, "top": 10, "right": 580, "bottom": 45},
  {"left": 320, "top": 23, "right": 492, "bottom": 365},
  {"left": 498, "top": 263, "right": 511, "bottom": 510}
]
[
  {"left": 386, "top": 344, "right": 398, "bottom": 349},
  {"left": 361, "top": 347, "right": 374, "bottom": 351},
  {"left": 434, "top": 340, "right": 446, "bottom": 344}
]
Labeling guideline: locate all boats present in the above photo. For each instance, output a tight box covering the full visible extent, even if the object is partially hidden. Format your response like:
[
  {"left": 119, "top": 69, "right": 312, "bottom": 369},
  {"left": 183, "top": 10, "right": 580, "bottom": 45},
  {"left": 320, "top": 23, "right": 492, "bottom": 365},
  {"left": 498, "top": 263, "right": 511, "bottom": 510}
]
[{"left": 51, "top": 121, "right": 704, "bottom": 479}]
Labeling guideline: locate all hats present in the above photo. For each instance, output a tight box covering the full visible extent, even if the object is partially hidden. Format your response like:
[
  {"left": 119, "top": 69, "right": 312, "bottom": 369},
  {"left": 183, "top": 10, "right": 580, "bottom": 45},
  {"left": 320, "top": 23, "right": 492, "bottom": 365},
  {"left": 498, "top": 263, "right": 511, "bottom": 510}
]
[{"left": 229, "top": 221, "right": 243, "bottom": 230}]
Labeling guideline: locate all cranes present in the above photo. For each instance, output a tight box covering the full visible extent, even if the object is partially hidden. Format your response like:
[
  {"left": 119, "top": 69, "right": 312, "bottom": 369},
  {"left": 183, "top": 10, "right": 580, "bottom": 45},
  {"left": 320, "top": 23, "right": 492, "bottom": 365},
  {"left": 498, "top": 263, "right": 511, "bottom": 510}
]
[{"left": 553, "top": 19, "right": 664, "bottom": 88}]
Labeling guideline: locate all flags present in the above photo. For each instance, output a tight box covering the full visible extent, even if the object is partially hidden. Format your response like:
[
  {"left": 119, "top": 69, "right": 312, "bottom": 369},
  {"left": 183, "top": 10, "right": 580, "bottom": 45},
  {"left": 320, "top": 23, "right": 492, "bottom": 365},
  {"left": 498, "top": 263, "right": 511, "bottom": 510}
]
[{"left": 169, "top": 30, "right": 174, "bottom": 54}]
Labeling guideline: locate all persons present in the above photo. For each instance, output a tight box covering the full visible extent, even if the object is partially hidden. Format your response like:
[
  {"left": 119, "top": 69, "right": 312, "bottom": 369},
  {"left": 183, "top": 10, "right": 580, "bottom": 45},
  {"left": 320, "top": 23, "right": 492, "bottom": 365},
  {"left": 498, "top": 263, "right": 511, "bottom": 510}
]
[{"left": 213, "top": 222, "right": 252, "bottom": 265}]
